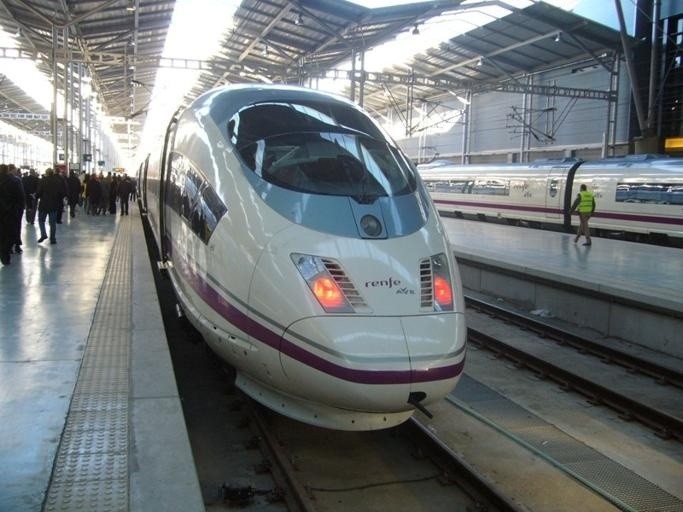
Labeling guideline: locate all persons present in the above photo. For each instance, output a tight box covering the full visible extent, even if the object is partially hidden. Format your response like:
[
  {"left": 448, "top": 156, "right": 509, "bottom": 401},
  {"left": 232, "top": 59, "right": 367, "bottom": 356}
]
[
  {"left": 573, "top": 243, "right": 591, "bottom": 266},
  {"left": 36, "top": 244, "right": 60, "bottom": 285},
  {"left": 0, "top": 163, "right": 136, "bottom": 267},
  {"left": 567, "top": 184, "right": 596, "bottom": 246}
]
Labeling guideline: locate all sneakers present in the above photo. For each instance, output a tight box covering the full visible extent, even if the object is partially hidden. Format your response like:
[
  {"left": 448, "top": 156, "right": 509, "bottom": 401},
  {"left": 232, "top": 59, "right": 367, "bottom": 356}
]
[
  {"left": 8, "top": 245, "right": 21, "bottom": 254},
  {"left": 38, "top": 235, "right": 55, "bottom": 244}
]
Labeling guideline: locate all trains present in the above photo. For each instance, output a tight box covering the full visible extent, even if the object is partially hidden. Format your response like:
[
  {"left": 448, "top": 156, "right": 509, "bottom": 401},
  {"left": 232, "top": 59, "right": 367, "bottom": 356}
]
[
  {"left": 413, "top": 155, "right": 683, "bottom": 244},
  {"left": 137, "top": 80, "right": 468, "bottom": 432}
]
